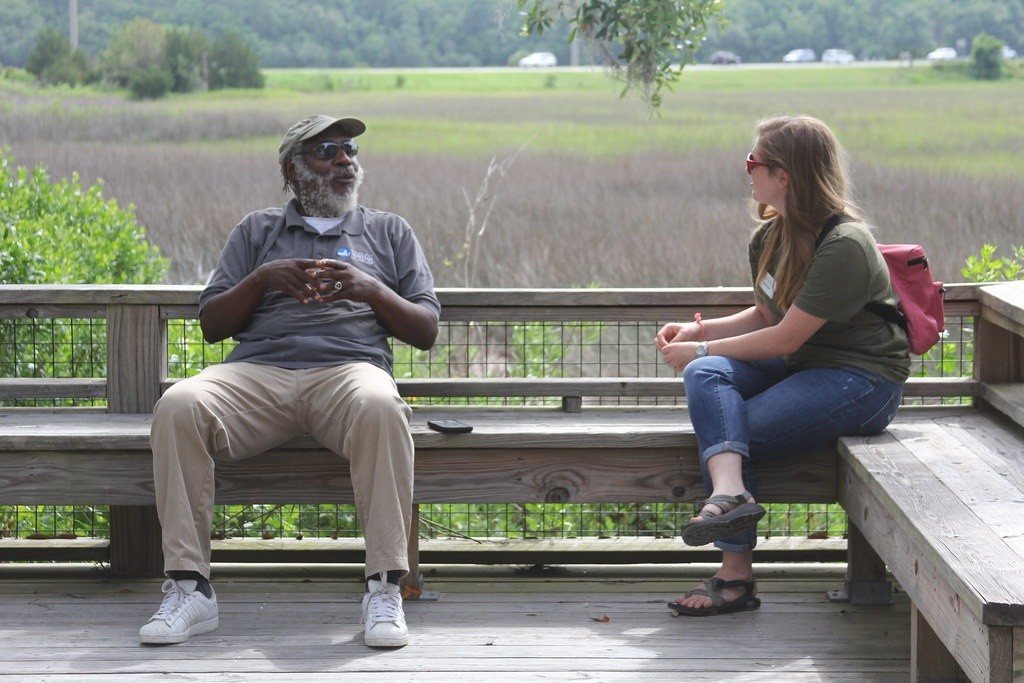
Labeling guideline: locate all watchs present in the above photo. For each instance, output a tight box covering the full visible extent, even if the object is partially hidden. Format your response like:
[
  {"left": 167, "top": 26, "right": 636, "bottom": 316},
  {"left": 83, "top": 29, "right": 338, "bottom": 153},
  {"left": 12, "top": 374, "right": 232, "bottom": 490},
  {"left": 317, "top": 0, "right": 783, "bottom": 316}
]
[{"left": 695, "top": 340, "right": 708, "bottom": 359}]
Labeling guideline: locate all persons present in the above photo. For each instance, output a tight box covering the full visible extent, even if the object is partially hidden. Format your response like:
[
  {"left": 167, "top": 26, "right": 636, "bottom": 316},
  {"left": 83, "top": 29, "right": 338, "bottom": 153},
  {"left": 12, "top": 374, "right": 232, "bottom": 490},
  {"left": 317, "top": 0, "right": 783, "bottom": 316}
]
[
  {"left": 654, "top": 114, "right": 911, "bottom": 617},
  {"left": 138, "top": 115, "right": 440, "bottom": 647}
]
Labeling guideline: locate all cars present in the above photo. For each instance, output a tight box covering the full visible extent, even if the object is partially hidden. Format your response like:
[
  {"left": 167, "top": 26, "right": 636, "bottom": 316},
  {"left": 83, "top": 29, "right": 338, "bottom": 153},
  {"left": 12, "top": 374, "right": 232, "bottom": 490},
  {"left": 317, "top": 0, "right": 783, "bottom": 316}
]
[
  {"left": 711, "top": 51, "right": 745, "bottom": 62},
  {"left": 822, "top": 49, "right": 855, "bottom": 63},
  {"left": 928, "top": 47, "right": 958, "bottom": 60},
  {"left": 783, "top": 48, "right": 816, "bottom": 63},
  {"left": 519, "top": 51, "right": 560, "bottom": 68}
]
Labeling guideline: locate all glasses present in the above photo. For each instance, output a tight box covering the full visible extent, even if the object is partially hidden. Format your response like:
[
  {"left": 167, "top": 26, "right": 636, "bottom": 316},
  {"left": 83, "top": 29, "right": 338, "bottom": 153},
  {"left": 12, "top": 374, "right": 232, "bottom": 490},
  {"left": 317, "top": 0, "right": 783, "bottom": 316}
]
[
  {"left": 294, "top": 140, "right": 360, "bottom": 160},
  {"left": 746, "top": 153, "right": 770, "bottom": 175}
]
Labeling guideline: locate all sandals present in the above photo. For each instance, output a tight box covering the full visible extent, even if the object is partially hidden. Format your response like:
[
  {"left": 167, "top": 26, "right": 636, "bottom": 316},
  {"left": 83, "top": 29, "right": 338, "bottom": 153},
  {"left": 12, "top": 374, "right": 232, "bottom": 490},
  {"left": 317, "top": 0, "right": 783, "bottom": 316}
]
[
  {"left": 680, "top": 490, "right": 766, "bottom": 546},
  {"left": 668, "top": 575, "right": 761, "bottom": 616}
]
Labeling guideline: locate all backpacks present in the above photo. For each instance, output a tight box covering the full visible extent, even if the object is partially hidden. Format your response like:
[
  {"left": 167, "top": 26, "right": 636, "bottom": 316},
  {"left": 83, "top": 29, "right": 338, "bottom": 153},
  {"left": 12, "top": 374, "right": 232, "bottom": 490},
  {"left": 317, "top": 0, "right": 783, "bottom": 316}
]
[{"left": 815, "top": 213, "right": 947, "bottom": 356}]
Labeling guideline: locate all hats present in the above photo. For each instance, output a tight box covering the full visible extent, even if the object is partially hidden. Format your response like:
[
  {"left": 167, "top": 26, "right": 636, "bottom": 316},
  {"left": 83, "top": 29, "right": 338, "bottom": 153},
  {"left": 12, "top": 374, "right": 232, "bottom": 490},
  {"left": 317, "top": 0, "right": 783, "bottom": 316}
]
[{"left": 278, "top": 115, "right": 367, "bottom": 164}]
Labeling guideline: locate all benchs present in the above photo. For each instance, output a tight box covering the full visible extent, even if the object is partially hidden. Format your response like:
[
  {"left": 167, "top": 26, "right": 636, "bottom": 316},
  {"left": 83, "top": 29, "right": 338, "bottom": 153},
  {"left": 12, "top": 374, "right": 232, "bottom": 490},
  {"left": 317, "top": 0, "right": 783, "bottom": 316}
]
[{"left": 0, "top": 285, "right": 1024, "bottom": 683}]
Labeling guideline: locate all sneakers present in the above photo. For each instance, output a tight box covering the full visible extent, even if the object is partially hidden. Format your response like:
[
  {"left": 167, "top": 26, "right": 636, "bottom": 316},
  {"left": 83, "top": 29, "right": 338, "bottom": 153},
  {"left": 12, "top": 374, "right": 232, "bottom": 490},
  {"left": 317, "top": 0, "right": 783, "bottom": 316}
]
[
  {"left": 360, "top": 571, "right": 409, "bottom": 647},
  {"left": 139, "top": 579, "right": 219, "bottom": 644}
]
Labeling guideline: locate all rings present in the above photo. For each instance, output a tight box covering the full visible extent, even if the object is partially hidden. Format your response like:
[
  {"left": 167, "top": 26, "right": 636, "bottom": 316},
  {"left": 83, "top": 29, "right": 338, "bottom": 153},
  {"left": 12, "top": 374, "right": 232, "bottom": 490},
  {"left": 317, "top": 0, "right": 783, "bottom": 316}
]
[{"left": 334, "top": 281, "right": 342, "bottom": 290}]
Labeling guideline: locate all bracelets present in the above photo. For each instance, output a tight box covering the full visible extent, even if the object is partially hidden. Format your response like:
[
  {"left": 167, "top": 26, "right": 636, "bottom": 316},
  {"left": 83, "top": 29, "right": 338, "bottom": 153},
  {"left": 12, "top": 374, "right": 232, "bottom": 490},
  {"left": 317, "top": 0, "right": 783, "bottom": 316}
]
[{"left": 694, "top": 313, "right": 704, "bottom": 342}]
